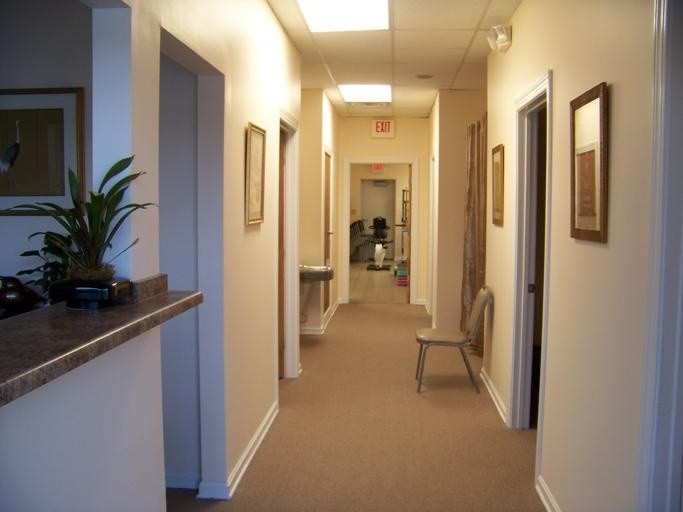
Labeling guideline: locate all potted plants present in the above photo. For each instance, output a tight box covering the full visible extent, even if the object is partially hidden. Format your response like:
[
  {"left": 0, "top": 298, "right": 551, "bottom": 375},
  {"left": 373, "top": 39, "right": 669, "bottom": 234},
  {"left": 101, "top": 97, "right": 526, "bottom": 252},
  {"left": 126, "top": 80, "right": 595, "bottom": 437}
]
[{"left": 5, "top": 154, "right": 162, "bottom": 310}]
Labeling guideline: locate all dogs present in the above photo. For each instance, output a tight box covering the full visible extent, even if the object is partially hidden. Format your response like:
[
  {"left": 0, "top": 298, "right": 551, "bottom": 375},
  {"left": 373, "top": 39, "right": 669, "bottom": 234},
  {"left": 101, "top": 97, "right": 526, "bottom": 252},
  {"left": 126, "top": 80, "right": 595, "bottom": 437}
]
[{"left": 373, "top": 243, "right": 386, "bottom": 268}]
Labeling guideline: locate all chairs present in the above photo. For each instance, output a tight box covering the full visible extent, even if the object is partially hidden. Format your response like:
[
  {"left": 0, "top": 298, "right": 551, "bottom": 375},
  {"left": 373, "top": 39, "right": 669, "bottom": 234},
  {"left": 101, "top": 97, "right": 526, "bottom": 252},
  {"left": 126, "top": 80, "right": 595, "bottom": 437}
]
[{"left": 414, "top": 286, "right": 489, "bottom": 394}]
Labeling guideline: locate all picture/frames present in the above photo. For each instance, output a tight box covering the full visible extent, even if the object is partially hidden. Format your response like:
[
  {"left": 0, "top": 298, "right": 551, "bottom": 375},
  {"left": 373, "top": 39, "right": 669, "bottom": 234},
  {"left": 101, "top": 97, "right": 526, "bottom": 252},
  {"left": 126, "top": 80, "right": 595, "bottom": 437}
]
[
  {"left": 245, "top": 122, "right": 267, "bottom": 227},
  {"left": 491, "top": 145, "right": 504, "bottom": 226},
  {"left": 569, "top": 82, "right": 609, "bottom": 244},
  {"left": 0, "top": 86, "right": 84, "bottom": 218}
]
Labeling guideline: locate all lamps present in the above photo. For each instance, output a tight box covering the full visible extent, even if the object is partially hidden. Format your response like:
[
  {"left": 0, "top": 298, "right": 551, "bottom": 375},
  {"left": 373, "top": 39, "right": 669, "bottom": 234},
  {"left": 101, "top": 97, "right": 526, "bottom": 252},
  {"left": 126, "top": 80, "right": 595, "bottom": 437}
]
[{"left": 484, "top": 24, "right": 512, "bottom": 54}]
